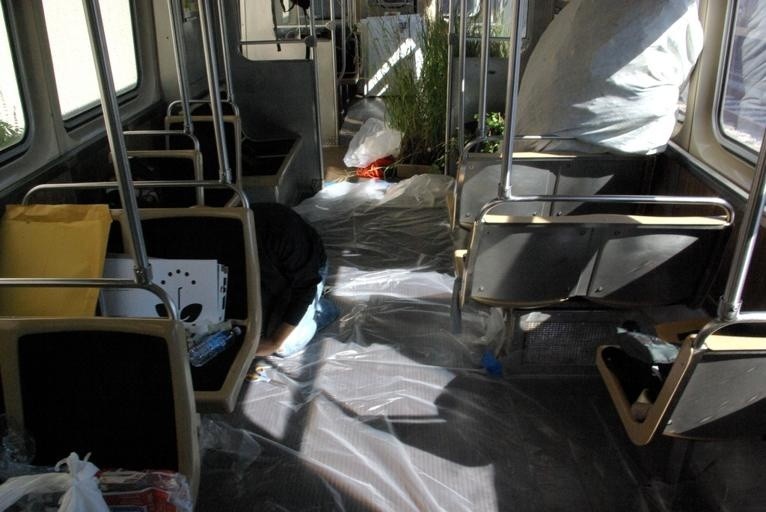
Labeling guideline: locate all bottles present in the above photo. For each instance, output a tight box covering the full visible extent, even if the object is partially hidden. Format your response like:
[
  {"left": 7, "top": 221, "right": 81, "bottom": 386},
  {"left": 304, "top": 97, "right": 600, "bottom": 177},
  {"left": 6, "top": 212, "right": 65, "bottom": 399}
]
[{"left": 189, "top": 326, "right": 242, "bottom": 368}]
